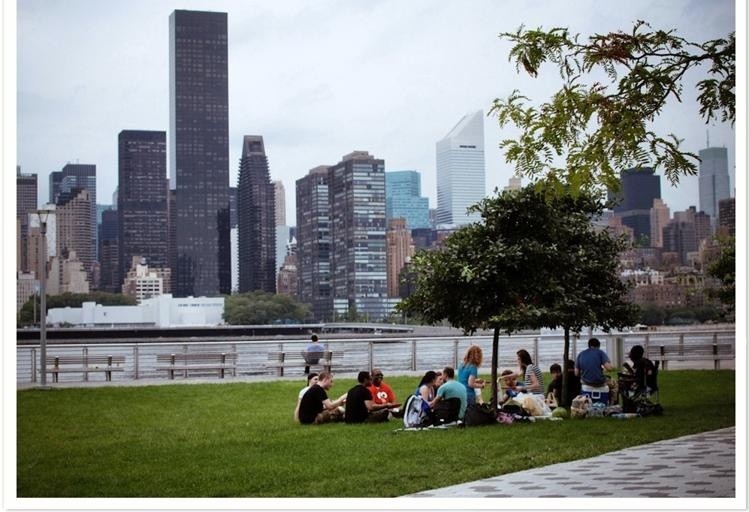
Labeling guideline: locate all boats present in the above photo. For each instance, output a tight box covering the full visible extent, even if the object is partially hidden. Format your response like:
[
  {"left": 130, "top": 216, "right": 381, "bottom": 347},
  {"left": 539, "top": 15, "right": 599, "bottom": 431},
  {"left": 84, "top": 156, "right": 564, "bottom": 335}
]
[{"left": 374, "top": 338, "right": 383, "bottom": 343}]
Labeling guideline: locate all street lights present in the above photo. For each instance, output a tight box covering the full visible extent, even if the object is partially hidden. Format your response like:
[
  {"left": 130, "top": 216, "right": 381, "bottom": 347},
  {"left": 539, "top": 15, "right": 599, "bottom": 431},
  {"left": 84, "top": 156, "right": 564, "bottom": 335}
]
[{"left": 35, "top": 202, "right": 51, "bottom": 386}]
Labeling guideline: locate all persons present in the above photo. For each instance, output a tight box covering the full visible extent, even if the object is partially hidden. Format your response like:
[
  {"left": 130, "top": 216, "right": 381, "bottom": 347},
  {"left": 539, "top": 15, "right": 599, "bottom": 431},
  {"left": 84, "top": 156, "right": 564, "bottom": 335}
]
[
  {"left": 303, "top": 335, "right": 326, "bottom": 375},
  {"left": 293, "top": 339, "right": 659, "bottom": 430}
]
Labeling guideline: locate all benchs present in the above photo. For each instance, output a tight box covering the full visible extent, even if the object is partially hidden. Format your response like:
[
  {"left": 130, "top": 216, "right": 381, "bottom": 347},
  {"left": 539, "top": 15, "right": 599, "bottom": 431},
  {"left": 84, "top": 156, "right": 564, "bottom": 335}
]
[
  {"left": 264, "top": 351, "right": 344, "bottom": 377},
  {"left": 644, "top": 344, "right": 736, "bottom": 371},
  {"left": 36, "top": 355, "right": 126, "bottom": 383},
  {"left": 153, "top": 353, "right": 238, "bottom": 380}
]
